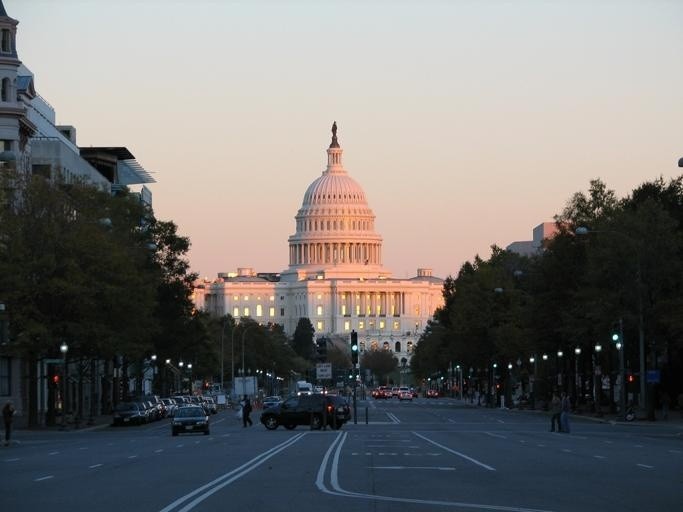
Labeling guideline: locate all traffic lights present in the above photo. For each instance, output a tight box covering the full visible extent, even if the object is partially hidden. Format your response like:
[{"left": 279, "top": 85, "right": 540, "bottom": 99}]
[
  {"left": 47, "top": 364, "right": 59, "bottom": 389},
  {"left": 351, "top": 331, "right": 358, "bottom": 365},
  {"left": 347, "top": 369, "right": 355, "bottom": 381},
  {"left": 627, "top": 373, "right": 636, "bottom": 382}
]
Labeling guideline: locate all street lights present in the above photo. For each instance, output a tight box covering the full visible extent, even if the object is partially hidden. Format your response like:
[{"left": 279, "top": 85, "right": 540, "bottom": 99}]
[
  {"left": 237, "top": 368, "right": 285, "bottom": 398},
  {"left": 59, "top": 341, "right": 71, "bottom": 427},
  {"left": 572, "top": 226, "right": 653, "bottom": 421},
  {"left": 203, "top": 379, "right": 209, "bottom": 390},
  {"left": 512, "top": 271, "right": 543, "bottom": 277},
  {"left": 149, "top": 354, "right": 193, "bottom": 398},
  {"left": 493, "top": 286, "right": 541, "bottom": 380},
  {"left": 428, "top": 330, "right": 627, "bottom": 422},
  {"left": 133, "top": 243, "right": 158, "bottom": 252},
  {"left": 76, "top": 216, "right": 112, "bottom": 229},
  {"left": 181, "top": 285, "right": 205, "bottom": 291}
]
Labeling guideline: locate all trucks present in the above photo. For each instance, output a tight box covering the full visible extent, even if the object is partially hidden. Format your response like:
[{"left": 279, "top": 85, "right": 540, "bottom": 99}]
[{"left": 291, "top": 380, "right": 327, "bottom": 397}]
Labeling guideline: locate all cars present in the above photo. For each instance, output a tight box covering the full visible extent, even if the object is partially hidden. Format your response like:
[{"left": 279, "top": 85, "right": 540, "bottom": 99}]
[
  {"left": 112, "top": 393, "right": 218, "bottom": 435},
  {"left": 371, "top": 385, "right": 439, "bottom": 399}
]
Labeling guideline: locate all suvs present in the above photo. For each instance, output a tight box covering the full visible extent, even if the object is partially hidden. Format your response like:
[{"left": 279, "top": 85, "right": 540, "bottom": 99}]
[
  {"left": 259, "top": 393, "right": 352, "bottom": 431},
  {"left": 263, "top": 396, "right": 280, "bottom": 410}
]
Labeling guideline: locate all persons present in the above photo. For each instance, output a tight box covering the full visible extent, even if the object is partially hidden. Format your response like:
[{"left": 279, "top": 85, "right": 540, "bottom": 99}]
[
  {"left": 485, "top": 391, "right": 493, "bottom": 408},
  {"left": 561, "top": 391, "right": 571, "bottom": 433},
  {"left": 551, "top": 389, "right": 560, "bottom": 431},
  {"left": 240, "top": 394, "right": 253, "bottom": 427},
  {"left": 2, "top": 399, "right": 16, "bottom": 447},
  {"left": 654, "top": 390, "right": 683, "bottom": 420}
]
